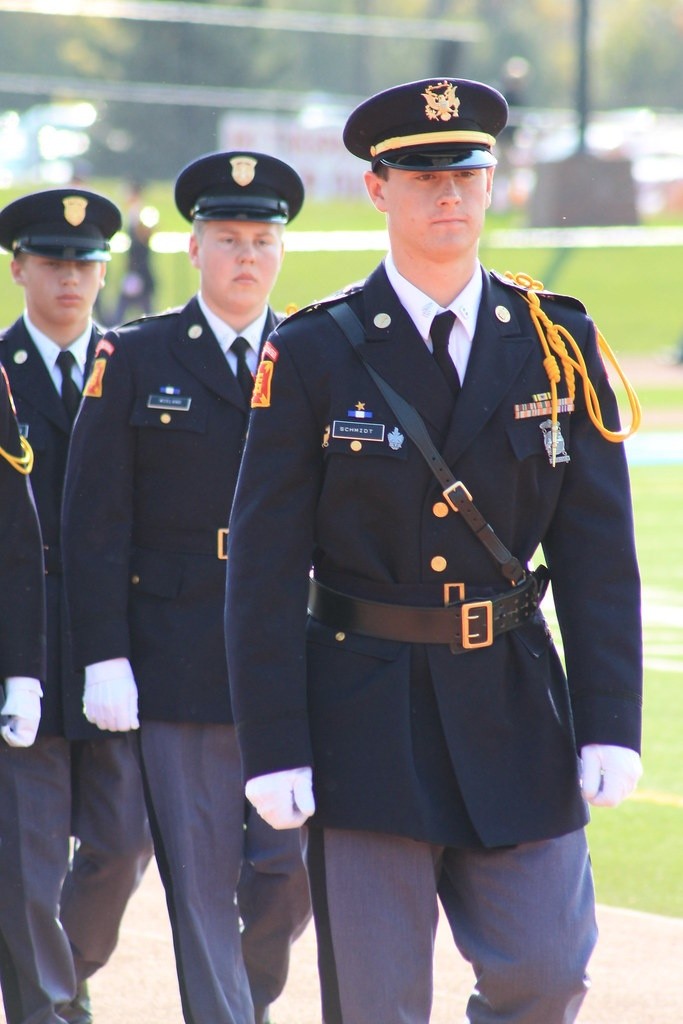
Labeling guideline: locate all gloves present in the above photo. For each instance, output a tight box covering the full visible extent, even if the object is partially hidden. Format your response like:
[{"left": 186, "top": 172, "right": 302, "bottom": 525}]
[
  {"left": 246, "top": 766, "right": 316, "bottom": 830},
  {"left": 82, "top": 658, "right": 140, "bottom": 732},
  {"left": 0, "top": 677, "right": 42, "bottom": 748},
  {"left": 579, "top": 744, "right": 643, "bottom": 808}
]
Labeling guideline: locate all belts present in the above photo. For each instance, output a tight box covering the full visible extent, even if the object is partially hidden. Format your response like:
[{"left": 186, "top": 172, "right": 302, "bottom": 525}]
[
  {"left": 306, "top": 563, "right": 551, "bottom": 650},
  {"left": 133, "top": 527, "right": 229, "bottom": 561}
]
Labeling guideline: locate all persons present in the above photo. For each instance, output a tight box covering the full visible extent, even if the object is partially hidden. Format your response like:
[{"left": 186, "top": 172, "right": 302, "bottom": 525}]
[
  {"left": 58, "top": 150, "right": 316, "bottom": 1024},
  {"left": 1, "top": 189, "right": 160, "bottom": 1024},
  {"left": 224, "top": 77, "right": 644, "bottom": 1024}
]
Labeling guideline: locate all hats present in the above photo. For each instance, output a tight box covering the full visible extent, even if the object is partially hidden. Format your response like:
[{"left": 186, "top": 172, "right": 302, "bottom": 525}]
[
  {"left": 0, "top": 189, "right": 123, "bottom": 262},
  {"left": 174, "top": 151, "right": 304, "bottom": 225},
  {"left": 341, "top": 78, "right": 508, "bottom": 172}
]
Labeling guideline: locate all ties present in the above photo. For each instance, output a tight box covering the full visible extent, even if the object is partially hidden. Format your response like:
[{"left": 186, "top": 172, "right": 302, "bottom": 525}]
[
  {"left": 430, "top": 310, "right": 461, "bottom": 399},
  {"left": 55, "top": 351, "right": 83, "bottom": 427},
  {"left": 230, "top": 335, "right": 254, "bottom": 404}
]
